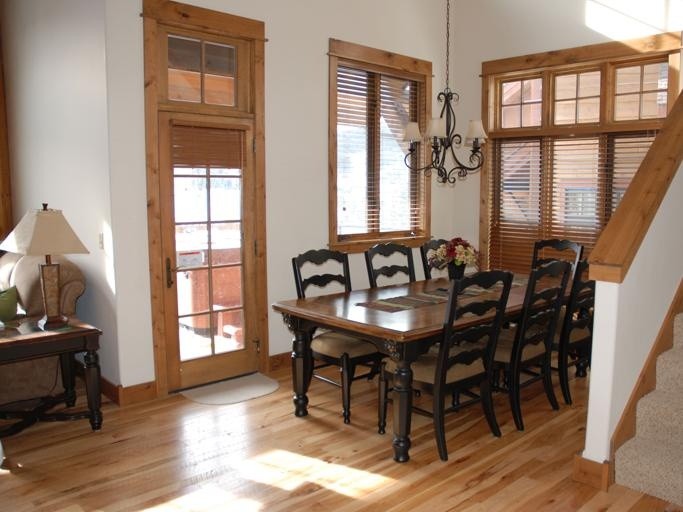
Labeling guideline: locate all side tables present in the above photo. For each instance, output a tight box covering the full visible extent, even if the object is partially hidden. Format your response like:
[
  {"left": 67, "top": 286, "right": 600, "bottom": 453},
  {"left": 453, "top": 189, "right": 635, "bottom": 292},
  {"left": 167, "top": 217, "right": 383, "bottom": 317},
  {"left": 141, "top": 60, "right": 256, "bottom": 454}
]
[{"left": 0, "top": 318, "right": 103, "bottom": 438}]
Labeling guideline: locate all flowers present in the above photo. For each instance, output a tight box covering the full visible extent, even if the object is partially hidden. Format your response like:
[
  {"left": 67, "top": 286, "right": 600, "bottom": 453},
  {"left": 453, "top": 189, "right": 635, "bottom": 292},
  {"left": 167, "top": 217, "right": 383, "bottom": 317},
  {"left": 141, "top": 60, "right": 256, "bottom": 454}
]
[{"left": 428, "top": 238, "right": 485, "bottom": 270}]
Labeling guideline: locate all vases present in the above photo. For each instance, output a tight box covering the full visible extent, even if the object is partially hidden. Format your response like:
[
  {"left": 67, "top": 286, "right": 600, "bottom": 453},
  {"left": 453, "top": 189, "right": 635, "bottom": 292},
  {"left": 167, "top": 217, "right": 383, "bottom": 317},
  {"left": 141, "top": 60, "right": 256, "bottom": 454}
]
[{"left": 448, "top": 260, "right": 466, "bottom": 280}]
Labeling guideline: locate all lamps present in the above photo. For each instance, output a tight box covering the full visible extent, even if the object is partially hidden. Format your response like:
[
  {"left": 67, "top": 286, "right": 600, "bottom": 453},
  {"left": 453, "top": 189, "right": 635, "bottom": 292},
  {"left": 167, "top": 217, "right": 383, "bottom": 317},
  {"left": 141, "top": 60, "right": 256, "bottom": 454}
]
[
  {"left": 403, "top": 0, "right": 490, "bottom": 183},
  {"left": 0, "top": 203, "right": 91, "bottom": 331}
]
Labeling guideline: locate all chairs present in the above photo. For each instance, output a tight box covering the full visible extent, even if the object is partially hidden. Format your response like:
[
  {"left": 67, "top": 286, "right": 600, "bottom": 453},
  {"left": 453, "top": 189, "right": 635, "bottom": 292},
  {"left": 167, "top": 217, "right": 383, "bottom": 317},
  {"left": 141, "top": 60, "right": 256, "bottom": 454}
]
[
  {"left": 364, "top": 242, "right": 416, "bottom": 289},
  {"left": 378, "top": 270, "right": 514, "bottom": 462},
  {"left": 512, "top": 257, "right": 596, "bottom": 406},
  {"left": 420, "top": 239, "right": 448, "bottom": 280},
  {"left": 430, "top": 261, "right": 573, "bottom": 431},
  {"left": 532, "top": 238, "right": 585, "bottom": 279},
  {"left": 292, "top": 249, "right": 387, "bottom": 424}
]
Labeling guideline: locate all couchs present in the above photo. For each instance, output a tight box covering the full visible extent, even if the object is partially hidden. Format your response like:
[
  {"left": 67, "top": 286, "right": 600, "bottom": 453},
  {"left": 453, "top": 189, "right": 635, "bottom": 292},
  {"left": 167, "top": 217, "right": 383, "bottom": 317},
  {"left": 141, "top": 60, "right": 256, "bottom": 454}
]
[{"left": 0, "top": 252, "right": 86, "bottom": 405}]
